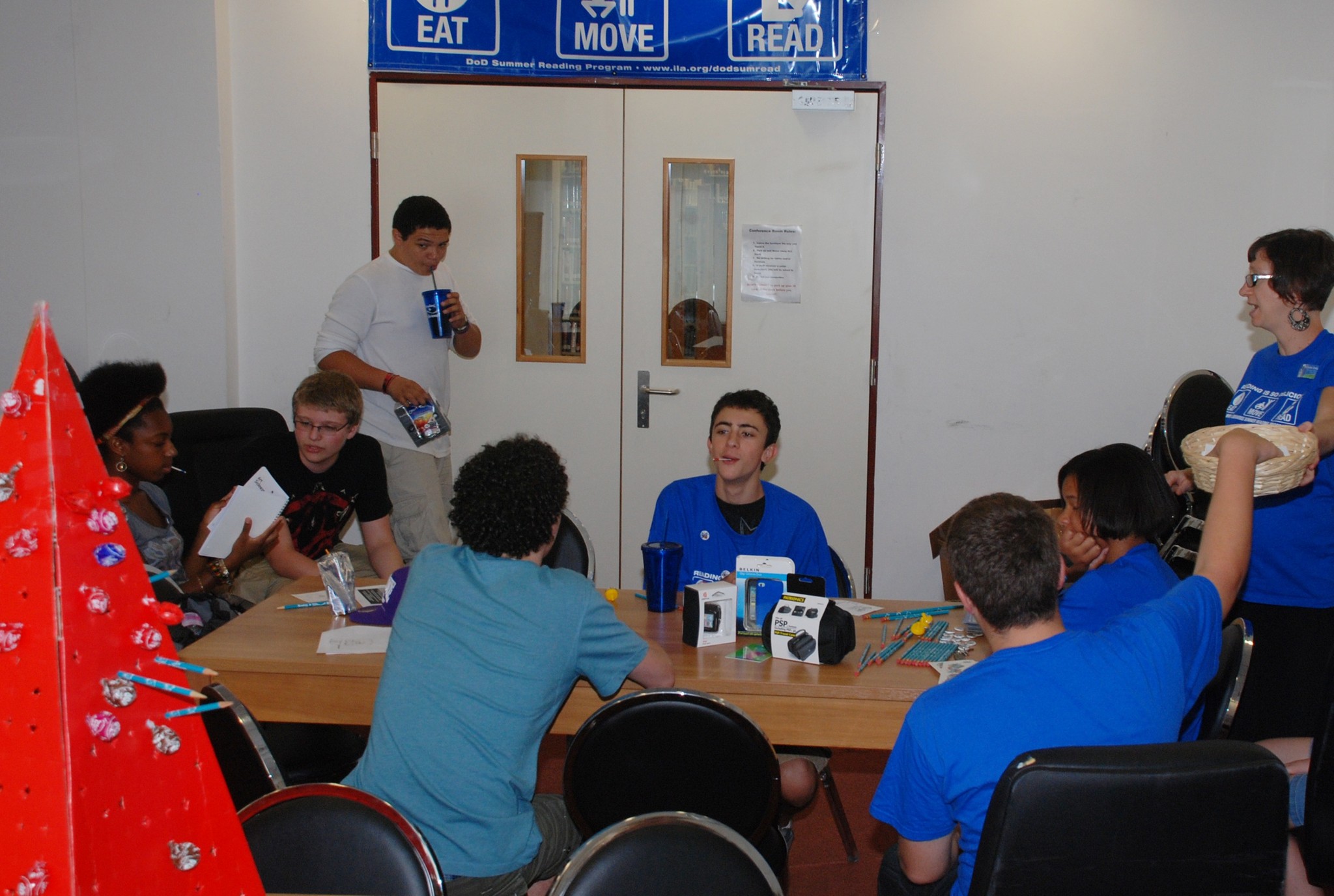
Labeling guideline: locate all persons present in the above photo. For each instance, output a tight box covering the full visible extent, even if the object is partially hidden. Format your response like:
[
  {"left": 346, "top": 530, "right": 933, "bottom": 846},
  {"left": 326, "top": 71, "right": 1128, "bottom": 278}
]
[
  {"left": 642, "top": 388, "right": 842, "bottom": 850},
  {"left": 871, "top": 427, "right": 1287, "bottom": 896},
  {"left": 337, "top": 432, "right": 678, "bottom": 896},
  {"left": 226, "top": 370, "right": 406, "bottom": 604},
  {"left": 1243, "top": 738, "right": 1334, "bottom": 896},
  {"left": 1053, "top": 440, "right": 1207, "bottom": 742},
  {"left": 312, "top": 195, "right": 483, "bottom": 570},
  {"left": 1164, "top": 229, "right": 1333, "bottom": 737},
  {"left": 73, "top": 360, "right": 287, "bottom": 650}
]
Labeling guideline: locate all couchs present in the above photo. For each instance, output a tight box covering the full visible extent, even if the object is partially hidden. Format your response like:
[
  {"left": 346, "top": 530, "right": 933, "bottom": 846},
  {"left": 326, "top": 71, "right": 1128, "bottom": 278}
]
[{"left": 144, "top": 405, "right": 294, "bottom": 553}]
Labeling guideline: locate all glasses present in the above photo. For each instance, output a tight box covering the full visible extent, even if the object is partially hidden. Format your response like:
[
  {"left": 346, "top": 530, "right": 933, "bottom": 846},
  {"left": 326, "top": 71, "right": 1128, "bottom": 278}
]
[
  {"left": 293, "top": 414, "right": 349, "bottom": 435},
  {"left": 1245, "top": 274, "right": 1274, "bottom": 287}
]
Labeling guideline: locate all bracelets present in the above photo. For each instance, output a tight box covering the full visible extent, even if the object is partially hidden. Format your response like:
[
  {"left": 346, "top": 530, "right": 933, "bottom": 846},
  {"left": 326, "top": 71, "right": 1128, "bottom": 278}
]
[
  {"left": 210, "top": 559, "right": 233, "bottom": 588},
  {"left": 382, "top": 373, "right": 400, "bottom": 394},
  {"left": 196, "top": 576, "right": 205, "bottom": 595}
]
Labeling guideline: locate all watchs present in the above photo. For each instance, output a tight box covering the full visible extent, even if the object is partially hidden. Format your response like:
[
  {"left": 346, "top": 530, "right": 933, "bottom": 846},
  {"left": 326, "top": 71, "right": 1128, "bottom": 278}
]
[{"left": 452, "top": 314, "right": 469, "bottom": 334}]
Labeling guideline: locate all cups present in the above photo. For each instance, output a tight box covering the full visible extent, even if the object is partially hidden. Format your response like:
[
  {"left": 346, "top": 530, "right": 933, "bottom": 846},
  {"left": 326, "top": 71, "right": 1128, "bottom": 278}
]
[
  {"left": 641, "top": 541, "right": 684, "bottom": 612},
  {"left": 422, "top": 289, "right": 455, "bottom": 340}
]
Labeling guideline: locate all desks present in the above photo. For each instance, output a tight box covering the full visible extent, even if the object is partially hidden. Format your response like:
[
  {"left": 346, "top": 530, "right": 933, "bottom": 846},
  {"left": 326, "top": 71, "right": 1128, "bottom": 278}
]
[{"left": 172, "top": 575, "right": 997, "bottom": 756}]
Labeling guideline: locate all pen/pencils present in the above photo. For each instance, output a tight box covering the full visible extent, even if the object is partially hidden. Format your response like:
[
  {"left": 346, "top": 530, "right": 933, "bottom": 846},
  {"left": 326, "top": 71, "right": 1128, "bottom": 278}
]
[
  {"left": 116, "top": 670, "right": 207, "bottom": 700},
  {"left": 155, "top": 656, "right": 220, "bottom": 677},
  {"left": 853, "top": 605, "right": 964, "bottom": 675},
  {"left": 277, "top": 599, "right": 329, "bottom": 610},
  {"left": 165, "top": 701, "right": 236, "bottom": 718},
  {"left": 149, "top": 569, "right": 178, "bottom": 584},
  {"left": 635, "top": 593, "right": 683, "bottom": 611}
]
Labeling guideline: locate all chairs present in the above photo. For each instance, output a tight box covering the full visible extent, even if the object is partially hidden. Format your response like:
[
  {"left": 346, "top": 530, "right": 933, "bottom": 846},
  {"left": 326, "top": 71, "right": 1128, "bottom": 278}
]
[
  {"left": 196, "top": 682, "right": 283, "bottom": 803},
  {"left": 1142, "top": 370, "right": 1242, "bottom": 590},
  {"left": 563, "top": 686, "right": 794, "bottom": 879},
  {"left": 537, "top": 507, "right": 602, "bottom": 585},
  {"left": 545, "top": 809, "right": 785, "bottom": 895},
  {"left": 967, "top": 740, "right": 1292, "bottom": 896},
  {"left": 227, "top": 781, "right": 445, "bottom": 896},
  {"left": 1195, "top": 615, "right": 1254, "bottom": 750},
  {"left": 779, "top": 546, "right": 863, "bottom": 863}
]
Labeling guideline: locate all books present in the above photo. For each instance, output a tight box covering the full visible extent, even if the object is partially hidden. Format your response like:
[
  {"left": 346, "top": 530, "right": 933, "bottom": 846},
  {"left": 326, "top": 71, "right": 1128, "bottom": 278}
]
[
  {"left": 198, "top": 465, "right": 292, "bottom": 559},
  {"left": 560, "top": 319, "right": 581, "bottom": 356}
]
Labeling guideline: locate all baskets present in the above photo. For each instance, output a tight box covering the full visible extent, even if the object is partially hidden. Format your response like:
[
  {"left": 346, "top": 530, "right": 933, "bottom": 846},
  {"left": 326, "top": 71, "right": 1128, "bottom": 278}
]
[{"left": 1180, "top": 423, "right": 1319, "bottom": 499}]
